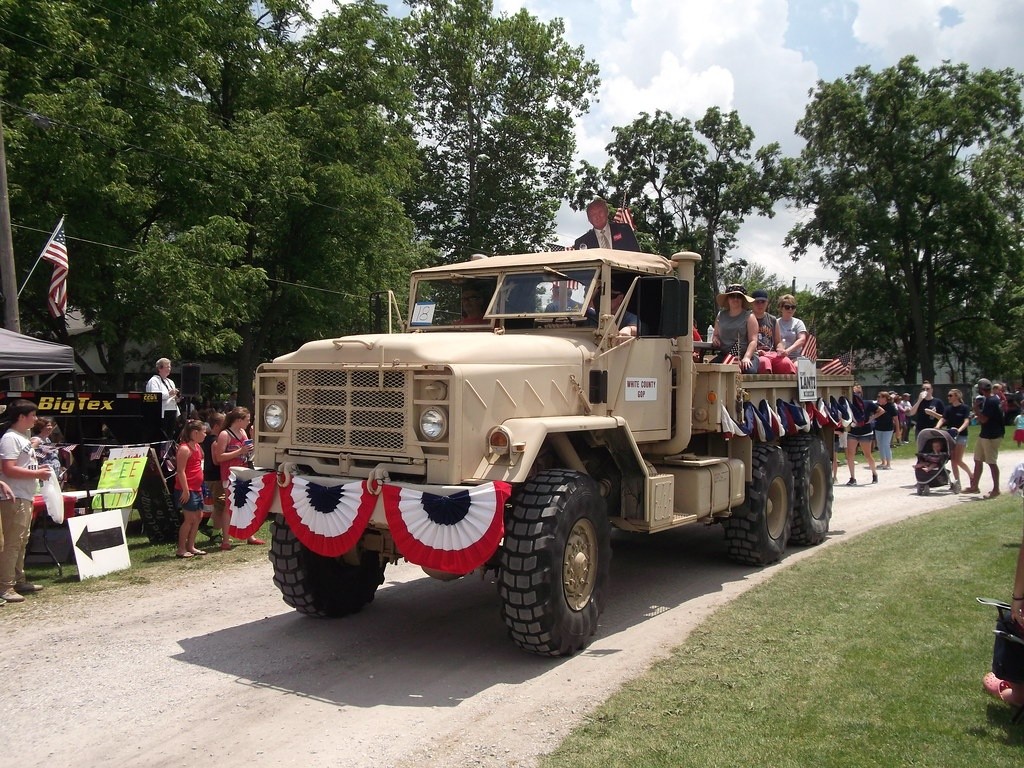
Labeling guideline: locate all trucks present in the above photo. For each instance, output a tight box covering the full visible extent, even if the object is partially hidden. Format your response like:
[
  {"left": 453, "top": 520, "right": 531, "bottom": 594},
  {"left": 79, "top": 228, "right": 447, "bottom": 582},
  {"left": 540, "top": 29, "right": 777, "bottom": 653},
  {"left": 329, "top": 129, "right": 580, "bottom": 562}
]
[{"left": 252, "top": 252, "right": 855, "bottom": 658}]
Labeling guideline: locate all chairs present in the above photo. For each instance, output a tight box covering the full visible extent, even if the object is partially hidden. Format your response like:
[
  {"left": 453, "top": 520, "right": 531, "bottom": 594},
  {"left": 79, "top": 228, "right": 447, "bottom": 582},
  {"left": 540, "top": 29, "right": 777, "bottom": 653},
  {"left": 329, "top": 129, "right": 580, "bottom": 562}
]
[{"left": 975, "top": 596, "right": 1024, "bottom": 725}]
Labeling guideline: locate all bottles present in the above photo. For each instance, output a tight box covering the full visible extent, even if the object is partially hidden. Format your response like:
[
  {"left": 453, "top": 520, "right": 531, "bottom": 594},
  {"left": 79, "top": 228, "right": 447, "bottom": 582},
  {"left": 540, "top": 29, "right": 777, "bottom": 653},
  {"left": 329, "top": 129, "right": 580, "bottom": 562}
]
[
  {"left": 782, "top": 338, "right": 786, "bottom": 349},
  {"left": 971, "top": 413, "right": 977, "bottom": 426},
  {"left": 707, "top": 325, "right": 714, "bottom": 343},
  {"left": 929, "top": 404, "right": 934, "bottom": 420}
]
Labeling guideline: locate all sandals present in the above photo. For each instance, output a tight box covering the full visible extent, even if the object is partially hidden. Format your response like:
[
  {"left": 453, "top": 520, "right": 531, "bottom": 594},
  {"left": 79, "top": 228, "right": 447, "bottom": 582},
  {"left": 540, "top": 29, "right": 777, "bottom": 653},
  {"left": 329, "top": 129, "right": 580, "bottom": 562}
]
[{"left": 983, "top": 671, "right": 1023, "bottom": 708}]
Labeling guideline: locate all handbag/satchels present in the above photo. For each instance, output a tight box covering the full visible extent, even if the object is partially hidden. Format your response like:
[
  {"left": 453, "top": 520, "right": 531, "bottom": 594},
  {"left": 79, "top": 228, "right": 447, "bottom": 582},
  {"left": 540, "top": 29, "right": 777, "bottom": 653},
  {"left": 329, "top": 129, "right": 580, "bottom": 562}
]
[{"left": 976, "top": 597, "right": 1024, "bottom": 682}]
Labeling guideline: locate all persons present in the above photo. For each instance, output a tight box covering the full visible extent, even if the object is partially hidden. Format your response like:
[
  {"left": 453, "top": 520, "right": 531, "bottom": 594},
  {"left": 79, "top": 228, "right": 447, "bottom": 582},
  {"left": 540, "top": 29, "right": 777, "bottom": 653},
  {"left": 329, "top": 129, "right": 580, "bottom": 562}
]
[
  {"left": 934, "top": 388, "right": 974, "bottom": 491},
  {"left": 844, "top": 385, "right": 885, "bottom": 486},
  {"left": 1011, "top": 535, "right": 1024, "bottom": 628},
  {"left": 909, "top": 379, "right": 945, "bottom": 441},
  {"left": 874, "top": 390, "right": 912, "bottom": 469},
  {"left": 912, "top": 440, "right": 943, "bottom": 472},
  {"left": 0, "top": 395, "right": 255, "bottom": 606},
  {"left": 145, "top": 358, "right": 182, "bottom": 424},
  {"left": 969, "top": 379, "right": 1024, "bottom": 499},
  {"left": 574, "top": 199, "right": 641, "bottom": 252},
  {"left": 461, "top": 279, "right": 804, "bottom": 374}
]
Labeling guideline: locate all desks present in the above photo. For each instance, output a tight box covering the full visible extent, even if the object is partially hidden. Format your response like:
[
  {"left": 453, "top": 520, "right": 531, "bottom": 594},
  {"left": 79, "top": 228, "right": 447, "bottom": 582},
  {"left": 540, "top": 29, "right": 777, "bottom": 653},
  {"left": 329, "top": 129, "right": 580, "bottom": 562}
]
[
  {"left": 22, "top": 488, "right": 133, "bottom": 576},
  {"left": 694, "top": 340, "right": 715, "bottom": 363}
]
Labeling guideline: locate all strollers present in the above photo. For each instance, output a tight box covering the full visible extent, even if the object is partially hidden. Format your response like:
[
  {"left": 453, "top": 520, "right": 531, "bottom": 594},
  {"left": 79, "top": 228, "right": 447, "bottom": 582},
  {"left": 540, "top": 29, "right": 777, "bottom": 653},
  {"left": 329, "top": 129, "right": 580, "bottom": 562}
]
[{"left": 914, "top": 427, "right": 959, "bottom": 495}]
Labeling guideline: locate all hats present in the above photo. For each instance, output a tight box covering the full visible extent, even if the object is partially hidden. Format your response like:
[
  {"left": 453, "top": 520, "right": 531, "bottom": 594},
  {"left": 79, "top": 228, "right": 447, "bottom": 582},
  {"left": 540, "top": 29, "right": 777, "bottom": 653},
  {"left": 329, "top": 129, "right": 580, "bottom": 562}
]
[
  {"left": 717, "top": 284, "right": 756, "bottom": 308},
  {"left": 889, "top": 391, "right": 898, "bottom": 395},
  {"left": 751, "top": 289, "right": 769, "bottom": 301},
  {"left": 903, "top": 393, "right": 911, "bottom": 397},
  {"left": 975, "top": 378, "right": 992, "bottom": 390}
]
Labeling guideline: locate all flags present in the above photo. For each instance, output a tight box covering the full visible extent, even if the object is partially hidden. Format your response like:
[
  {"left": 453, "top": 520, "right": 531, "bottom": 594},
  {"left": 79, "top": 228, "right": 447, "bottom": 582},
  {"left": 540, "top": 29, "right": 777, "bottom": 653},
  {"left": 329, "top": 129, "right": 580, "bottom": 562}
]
[
  {"left": 41, "top": 219, "right": 69, "bottom": 318},
  {"left": 614, "top": 195, "right": 636, "bottom": 233},
  {"left": 819, "top": 355, "right": 854, "bottom": 376},
  {"left": 800, "top": 320, "right": 816, "bottom": 364},
  {"left": 723, "top": 340, "right": 738, "bottom": 365}
]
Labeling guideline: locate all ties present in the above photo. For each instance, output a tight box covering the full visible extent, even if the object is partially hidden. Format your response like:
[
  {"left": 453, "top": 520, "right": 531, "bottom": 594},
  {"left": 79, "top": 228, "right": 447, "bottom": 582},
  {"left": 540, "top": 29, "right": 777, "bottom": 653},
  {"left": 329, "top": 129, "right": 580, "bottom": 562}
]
[{"left": 601, "top": 230, "right": 610, "bottom": 249}]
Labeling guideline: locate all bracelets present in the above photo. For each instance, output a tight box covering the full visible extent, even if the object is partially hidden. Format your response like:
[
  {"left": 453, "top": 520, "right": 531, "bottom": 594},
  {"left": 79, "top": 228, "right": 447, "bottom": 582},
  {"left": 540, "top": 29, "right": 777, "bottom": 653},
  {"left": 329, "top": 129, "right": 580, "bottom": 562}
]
[{"left": 1011, "top": 592, "right": 1023, "bottom": 601}]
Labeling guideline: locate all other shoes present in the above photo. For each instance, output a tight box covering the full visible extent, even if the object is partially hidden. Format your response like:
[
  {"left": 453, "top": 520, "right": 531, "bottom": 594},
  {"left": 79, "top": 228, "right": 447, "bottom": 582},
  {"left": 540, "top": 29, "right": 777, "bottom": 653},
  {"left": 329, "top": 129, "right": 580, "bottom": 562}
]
[
  {"left": 984, "top": 489, "right": 1000, "bottom": 499},
  {"left": 872, "top": 439, "right": 909, "bottom": 452},
  {"left": 877, "top": 464, "right": 884, "bottom": 468},
  {"left": 177, "top": 552, "right": 194, "bottom": 558},
  {"left": 872, "top": 474, "right": 878, "bottom": 483},
  {"left": 846, "top": 478, "right": 856, "bottom": 487},
  {"left": 14, "top": 581, "right": 43, "bottom": 592},
  {"left": 883, "top": 465, "right": 891, "bottom": 470},
  {"left": 970, "top": 473, "right": 974, "bottom": 486},
  {"left": 965, "top": 486, "right": 980, "bottom": 494},
  {"left": 220, "top": 543, "right": 230, "bottom": 550},
  {"left": 247, "top": 537, "right": 266, "bottom": 545},
  {"left": 2, "top": 593, "right": 25, "bottom": 602},
  {"left": 188, "top": 549, "right": 207, "bottom": 555}
]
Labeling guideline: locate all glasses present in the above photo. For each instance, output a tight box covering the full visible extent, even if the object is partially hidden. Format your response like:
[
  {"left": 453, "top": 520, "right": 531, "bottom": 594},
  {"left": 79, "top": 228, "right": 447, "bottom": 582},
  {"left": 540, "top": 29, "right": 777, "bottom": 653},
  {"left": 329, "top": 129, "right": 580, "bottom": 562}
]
[
  {"left": 785, "top": 305, "right": 796, "bottom": 310},
  {"left": 611, "top": 293, "right": 622, "bottom": 299},
  {"left": 947, "top": 394, "right": 953, "bottom": 398}
]
